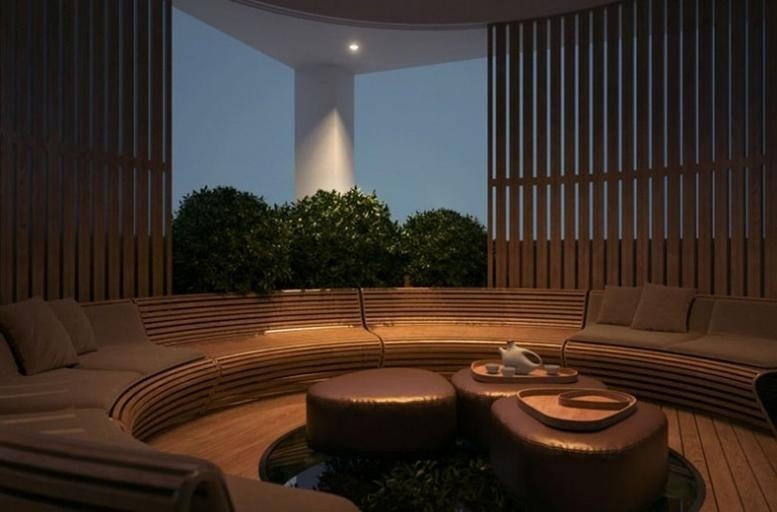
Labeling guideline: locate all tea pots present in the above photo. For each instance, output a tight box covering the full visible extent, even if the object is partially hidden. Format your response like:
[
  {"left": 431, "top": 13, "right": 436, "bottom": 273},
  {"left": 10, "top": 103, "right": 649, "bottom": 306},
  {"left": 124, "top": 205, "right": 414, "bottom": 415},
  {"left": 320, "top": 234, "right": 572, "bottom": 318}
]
[{"left": 499, "top": 339, "right": 542, "bottom": 375}]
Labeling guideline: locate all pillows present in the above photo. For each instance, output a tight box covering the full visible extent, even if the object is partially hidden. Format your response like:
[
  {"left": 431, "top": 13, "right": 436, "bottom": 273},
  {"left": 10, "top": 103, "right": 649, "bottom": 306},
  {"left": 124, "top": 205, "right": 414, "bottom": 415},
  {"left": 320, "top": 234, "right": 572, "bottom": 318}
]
[{"left": 592, "top": 281, "right": 696, "bottom": 334}]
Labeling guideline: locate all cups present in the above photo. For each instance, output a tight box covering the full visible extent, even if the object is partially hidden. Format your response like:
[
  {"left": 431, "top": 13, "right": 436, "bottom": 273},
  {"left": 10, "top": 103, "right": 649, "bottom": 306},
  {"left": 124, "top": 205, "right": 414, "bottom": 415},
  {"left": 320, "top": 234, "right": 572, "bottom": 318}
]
[
  {"left": 502, "top": 367, "right": 515, "bottom": 376},
  {"left": 486, "top": 363, "right": 499, "bottom": 374},
  {"left": 544, "top": 364, "right": 561, "bottom": 375}
]
